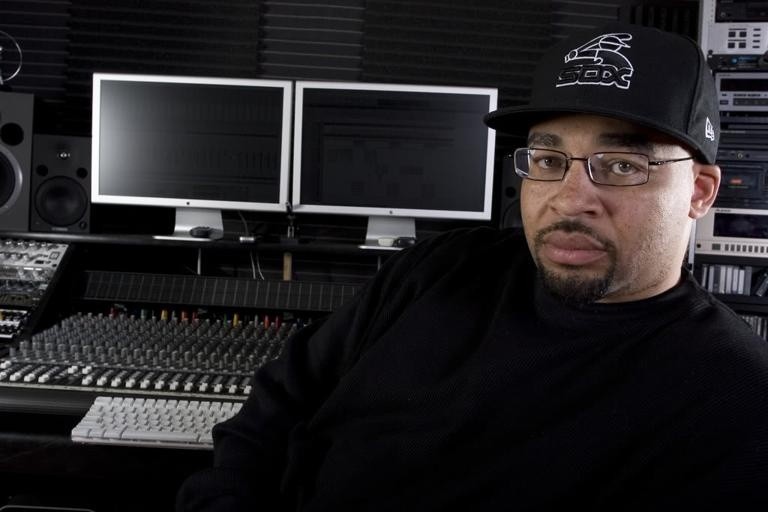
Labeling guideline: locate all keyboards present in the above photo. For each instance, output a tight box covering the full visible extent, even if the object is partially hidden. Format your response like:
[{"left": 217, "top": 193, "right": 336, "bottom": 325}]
[{"left": 71, "top": 396, "right": 244, "bottom": 452}]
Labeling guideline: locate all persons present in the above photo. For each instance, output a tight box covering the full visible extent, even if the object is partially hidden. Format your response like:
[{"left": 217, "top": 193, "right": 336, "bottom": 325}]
[{"left": 176, "top": 26, "right": 766, "bottom": 511}]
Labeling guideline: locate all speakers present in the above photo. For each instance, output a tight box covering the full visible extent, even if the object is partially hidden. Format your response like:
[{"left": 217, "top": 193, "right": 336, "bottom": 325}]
[
  {"left": 499, "top": 157, "right": 523, "bottom": 229},
  {"left": 29, "top": 135, "right": 90, "bottom": 233},
  {"left": 0, "top": 92, "right": 34, "bottom": 233}
]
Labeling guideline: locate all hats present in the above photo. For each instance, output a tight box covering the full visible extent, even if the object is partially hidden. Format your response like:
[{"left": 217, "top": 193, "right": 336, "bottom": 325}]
[{"left": 483, "top": 24, "right": 722, "bottom": 163}]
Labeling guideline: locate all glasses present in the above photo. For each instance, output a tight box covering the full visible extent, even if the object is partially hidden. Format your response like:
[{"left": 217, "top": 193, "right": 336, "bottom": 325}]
[{"left": 504, "top": 146, "right": 698, "bottom": 187}]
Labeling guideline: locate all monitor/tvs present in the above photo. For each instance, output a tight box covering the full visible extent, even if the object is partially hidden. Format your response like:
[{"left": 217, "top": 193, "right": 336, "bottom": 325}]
[
  {"left": 292, "top": 79, "right": 499, "bottom": 251},
  {"left": 90, "top": 71, "right": 293, "bottom": 243}
]
